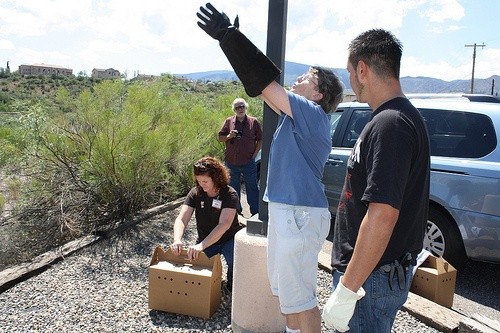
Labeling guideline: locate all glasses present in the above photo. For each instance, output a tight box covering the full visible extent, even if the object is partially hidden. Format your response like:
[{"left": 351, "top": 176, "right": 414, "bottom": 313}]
[{"left": 235, "top": 106, "right": 244, "bottom": 110}]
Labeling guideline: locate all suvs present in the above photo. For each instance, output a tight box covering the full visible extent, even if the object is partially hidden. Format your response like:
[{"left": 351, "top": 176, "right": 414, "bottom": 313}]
[{"left": 255, "top": 93, "right": 500, "bottom": 270}]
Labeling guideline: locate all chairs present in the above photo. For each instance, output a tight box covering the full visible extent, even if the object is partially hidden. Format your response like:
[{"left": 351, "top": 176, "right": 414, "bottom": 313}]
[
  {"left": 345, "top": 116, "right": 371, "bottom": 148},
  {"left": 456, "top": 125, "right": 481, "bottom": 159}
]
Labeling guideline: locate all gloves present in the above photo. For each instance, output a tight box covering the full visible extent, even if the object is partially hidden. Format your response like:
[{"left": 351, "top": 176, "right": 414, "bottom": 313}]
[
  {"left": 321, "top": 276, "right": 366, "bottom": 332},
  {"left": 197, "top": 3, "right": 281, "bottom": 97}
]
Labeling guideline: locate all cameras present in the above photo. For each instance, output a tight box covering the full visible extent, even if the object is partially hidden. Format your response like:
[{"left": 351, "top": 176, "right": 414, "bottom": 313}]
[{"left": 234, "top": 130, "right": 242, "bottom": 139}]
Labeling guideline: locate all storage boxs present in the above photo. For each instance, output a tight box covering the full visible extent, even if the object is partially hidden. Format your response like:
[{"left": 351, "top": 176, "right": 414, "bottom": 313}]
[
  {"left": 149, "top": 245, "right": 222, "bottom": 319},
  {"left": 409, "top": 251, "right": 457, "bottom": 310}
]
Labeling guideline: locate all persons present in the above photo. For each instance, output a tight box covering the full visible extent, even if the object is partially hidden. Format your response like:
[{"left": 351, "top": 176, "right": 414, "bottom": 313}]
[
  {"left": 321, "top": 28, "right": 433, "bottom": 333},
  {"left": 170, "top": 156, "right": 247, "bottom": 292},
  {"left": 217, "top": 97, "right": 262, "bottom": 218},
  {"left": 195, "top": 1, "right": 345, "bottom": 332}
]
